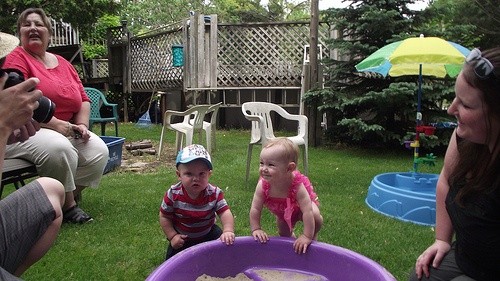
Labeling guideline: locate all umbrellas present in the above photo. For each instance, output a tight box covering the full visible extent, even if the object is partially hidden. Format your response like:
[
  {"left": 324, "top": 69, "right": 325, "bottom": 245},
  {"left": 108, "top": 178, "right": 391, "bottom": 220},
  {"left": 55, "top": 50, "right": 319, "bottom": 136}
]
[{"left": 354, "top": 33, "right": 472, "bottom": 172}]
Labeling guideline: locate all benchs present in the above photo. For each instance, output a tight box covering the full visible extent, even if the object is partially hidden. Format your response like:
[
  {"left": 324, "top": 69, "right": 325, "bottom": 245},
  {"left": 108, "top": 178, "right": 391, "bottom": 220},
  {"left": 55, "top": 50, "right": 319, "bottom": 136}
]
[{"left": 0, "top": 158, "right": 39, "bottom": 202}]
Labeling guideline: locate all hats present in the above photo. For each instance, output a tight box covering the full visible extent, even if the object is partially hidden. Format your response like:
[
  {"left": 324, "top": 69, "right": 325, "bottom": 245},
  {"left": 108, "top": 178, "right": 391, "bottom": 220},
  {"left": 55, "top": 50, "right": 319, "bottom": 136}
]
[{"left": 176, "top": 144, "right": 213, "bottom": 170}]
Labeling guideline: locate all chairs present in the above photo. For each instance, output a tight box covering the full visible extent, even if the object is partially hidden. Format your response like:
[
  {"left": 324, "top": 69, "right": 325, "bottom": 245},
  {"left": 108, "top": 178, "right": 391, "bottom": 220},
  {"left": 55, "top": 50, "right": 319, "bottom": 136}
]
[
  {"left": 190, "top": 101, "right": 223, "bottom": 154},
  {"left": 83, "top": 87, "right": 118, "bottom": 136},
  {"left": 241, "top": 101, "right": 309, "bottom": 183},
  {"left": 156, "top": 105, "right": 211, "bottom": 161}
]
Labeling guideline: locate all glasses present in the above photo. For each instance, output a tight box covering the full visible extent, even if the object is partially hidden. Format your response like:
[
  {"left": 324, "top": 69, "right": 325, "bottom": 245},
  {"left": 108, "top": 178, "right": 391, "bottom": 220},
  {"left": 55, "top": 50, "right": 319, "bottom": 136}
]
[{"left": 466, "top": 48, "right": 494, "bottom": 79}]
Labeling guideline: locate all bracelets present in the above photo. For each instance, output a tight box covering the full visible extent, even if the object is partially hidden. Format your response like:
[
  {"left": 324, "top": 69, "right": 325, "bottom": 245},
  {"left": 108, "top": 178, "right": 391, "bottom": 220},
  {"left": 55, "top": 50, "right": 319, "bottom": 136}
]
[{"left": 75, "top": 122, "right": 90, "bottom": 128}]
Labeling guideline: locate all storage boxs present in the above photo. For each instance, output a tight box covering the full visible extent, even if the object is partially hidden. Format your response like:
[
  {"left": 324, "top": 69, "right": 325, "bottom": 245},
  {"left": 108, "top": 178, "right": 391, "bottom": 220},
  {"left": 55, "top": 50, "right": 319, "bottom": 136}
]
[{"left": 99, "top": 136, "right": 124, "bottom": 175}]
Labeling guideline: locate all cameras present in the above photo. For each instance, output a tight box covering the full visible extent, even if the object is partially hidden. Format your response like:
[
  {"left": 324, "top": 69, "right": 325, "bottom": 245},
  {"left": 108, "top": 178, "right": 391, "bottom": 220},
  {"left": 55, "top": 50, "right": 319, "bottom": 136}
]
[{"left": 4, "top": 71, "right": 56, "bottom": 123}]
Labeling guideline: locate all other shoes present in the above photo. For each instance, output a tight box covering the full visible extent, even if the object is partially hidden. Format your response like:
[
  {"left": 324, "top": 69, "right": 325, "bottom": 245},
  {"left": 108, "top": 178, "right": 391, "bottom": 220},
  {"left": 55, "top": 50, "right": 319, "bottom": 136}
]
[{"left": 63, "top": 204, "right": 94, "bottom": 227}]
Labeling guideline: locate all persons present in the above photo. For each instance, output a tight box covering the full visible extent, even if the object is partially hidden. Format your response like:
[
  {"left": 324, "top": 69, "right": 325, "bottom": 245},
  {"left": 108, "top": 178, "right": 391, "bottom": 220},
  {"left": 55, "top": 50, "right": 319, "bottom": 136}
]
[
  {"left": 408, "top": 49, "right": 496, "bottom": 281},
  {"left": 250, "top": 138, "right": 324, "bottom": 254},
  {"left": 0, "top": 7, "right": 109, "bottom": 225},
  {"left": 0, "top": 69, "right": 65, "bottom": 281},
  {"left": 160, "top": 145, "right": 235, "bottom": 260}
]
